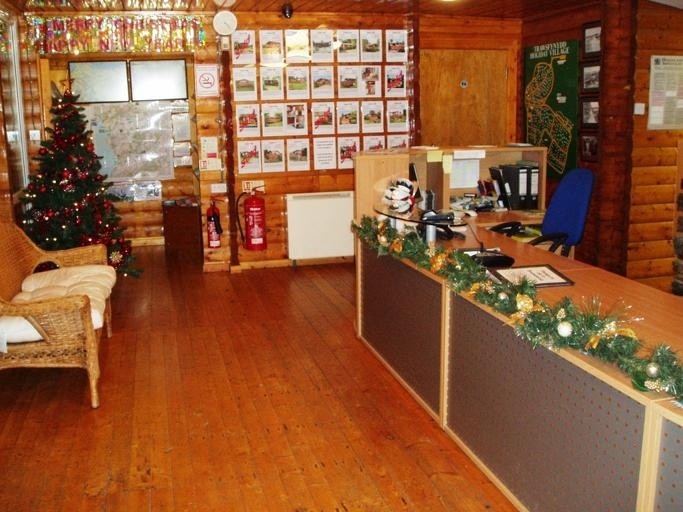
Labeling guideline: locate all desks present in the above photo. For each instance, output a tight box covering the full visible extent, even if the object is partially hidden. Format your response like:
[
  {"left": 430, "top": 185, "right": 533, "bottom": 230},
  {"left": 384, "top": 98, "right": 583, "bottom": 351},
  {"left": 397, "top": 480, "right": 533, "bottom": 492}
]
[{"left": 351, "top": 144, "right": 682, "bottom": 512}]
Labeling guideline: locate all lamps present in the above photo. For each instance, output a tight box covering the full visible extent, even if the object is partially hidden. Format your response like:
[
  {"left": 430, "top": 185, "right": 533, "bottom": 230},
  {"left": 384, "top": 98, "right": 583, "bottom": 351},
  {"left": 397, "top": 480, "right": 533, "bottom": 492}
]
[{"left": 212, "top": 8, "right": 237, "bottom": 35}]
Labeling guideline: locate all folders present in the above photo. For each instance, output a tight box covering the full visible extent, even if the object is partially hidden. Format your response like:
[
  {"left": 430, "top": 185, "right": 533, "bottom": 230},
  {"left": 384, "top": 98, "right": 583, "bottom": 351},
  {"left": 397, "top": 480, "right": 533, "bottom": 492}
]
[{"left": 488, "top": 164, "right": 540, "bottom": 212}]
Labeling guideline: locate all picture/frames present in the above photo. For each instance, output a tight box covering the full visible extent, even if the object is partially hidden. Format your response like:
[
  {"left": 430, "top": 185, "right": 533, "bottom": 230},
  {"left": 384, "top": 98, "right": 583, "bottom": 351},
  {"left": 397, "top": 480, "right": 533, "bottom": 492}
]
[
  {"left": 236, "top": 103, "right": 261, "bottom": 138},
  {"left": 311, "top": 137, "right": 336, "bottom": 171},
  {"left": 523, "top": 37, "right": 578, "bottom": 181},
  {"left": 386, "top": 100, "right": 411, "bottom": 133},
  {"left": 647, "top": 54, "right": 683, "bottom": 131},
  {"left": 336, "top": 136, "right": 362, "bottom": 169},
  {"left": 384, "top": 65, "right": 407, "bottom": 99},
  {"left": 336, "top": 29, "right": 360, "bottom": 62},
  {"left": 385, "top": 28, "right": 409, "bottom": 62},
  {"left": 236, "top": 141, "right": 262, "bottom": 175},
  {"left": 286, "top": 66, "right": 311, "bottom": 99},
  {"left": 579, "top": 97, "right": 603, "bottom": 133},
  {"left": 580, "top": 64, "right": 603, "bottom": 94},
  {"left": 310, "top": 65, "right": 335, "bottom": 100},
  {"left": 310, "top": 27, "right": 335, "bottom": 63},
  {"left": 259, "top": 29, "right": 284, "bottom": 67},
  {"left": 230, "top": 30, "right": 256, "bottom": 65},
  {"left": 579, "top": 19, "right": 603, "bottom": 62},
  {"left": 195, "top": 63, "right": 219, "bottom": 97},
  {"left": 336, "top": 101, "right": 360, "bottom": 134},
  {"left": 337, "top": 65, "right": 382, "bottom": 99},
  {"left": 360, "top": 100, "right": 384, "bottom": 134},
  {"left": 285, "top": 28, "right": 310, "bottom": 63},
  {"left": 259, "top": 67, "right": 284, "bottom": 101},
  {"left": 232, "top": 68, "right": 258, "bottom": 101},
  {"left": 359, "top": 28, "right": 382, "bottom": 62},
  {"left": 311, "top": 101, "right": 335, "bottom": 135},
  {"left": 362, "top": 135, "right": 385, "bottom": 153},
  {"left": 285, "top": 137, "right": 311, "bottom": 172},
  {"left": 386, "top": 133, "right": 411, "bottom": 151},
  {"left": 200, "top": 136, "right": 221, "bottom": 171},
  {"left": 260, "top": 101, "right": 309, "bottom": 137},
  {"left": 259, "top": 138, "right": 286, "bottom": 173},
  {"left": 577, "top": 131, "right": 602, "bottom": 170}
]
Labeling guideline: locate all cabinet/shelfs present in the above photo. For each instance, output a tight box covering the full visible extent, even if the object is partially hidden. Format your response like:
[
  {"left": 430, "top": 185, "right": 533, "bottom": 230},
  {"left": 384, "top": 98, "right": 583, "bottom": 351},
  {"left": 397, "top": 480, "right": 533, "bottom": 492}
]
[{"left": 162, "top": 199, "right": 201, "bottom": 277}]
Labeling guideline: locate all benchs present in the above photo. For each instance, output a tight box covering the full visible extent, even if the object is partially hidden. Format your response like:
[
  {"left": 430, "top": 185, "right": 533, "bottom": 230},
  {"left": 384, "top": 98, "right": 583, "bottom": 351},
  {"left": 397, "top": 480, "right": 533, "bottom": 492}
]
[{"left": 1, "top": 222, "right": 112, "bottom": 410}]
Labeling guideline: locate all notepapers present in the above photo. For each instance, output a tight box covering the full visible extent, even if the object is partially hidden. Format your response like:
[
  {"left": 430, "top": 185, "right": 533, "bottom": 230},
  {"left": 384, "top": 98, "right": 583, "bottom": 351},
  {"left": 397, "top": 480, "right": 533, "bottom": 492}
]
[
  {"left": 443, "top": 155, "right": 453, "bottom": 174},
  {"left": 427, "top": 151, "right": 443, "bottom": 163}
]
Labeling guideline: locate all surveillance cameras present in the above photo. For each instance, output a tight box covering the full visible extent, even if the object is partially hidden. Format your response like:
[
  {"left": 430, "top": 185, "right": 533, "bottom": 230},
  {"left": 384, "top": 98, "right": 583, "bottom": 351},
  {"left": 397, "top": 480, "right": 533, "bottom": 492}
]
[{"left": 282, "top": 4, "right": 293, "bottom": 19}]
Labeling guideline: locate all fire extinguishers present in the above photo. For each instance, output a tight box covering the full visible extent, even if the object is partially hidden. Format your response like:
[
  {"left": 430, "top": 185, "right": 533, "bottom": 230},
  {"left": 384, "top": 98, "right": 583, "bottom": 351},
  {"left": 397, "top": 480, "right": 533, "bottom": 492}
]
[
  {"left": 236, "top": 184, "right": 268, "bottom": 251},
  {"left": 206, "top": 196, "right": 225, "bottom": 249}
]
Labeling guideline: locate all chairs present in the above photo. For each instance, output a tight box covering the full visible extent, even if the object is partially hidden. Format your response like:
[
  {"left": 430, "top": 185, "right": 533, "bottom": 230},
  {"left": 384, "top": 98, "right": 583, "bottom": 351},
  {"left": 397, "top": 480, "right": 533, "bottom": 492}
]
[{"left": 529, "top": 166, "right": 594, "bottom": 257}]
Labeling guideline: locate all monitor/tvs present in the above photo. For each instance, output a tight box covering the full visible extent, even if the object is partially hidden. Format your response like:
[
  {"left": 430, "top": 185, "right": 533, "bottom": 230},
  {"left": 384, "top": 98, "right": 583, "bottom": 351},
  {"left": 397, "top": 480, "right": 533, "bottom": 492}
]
[{"left": 409, "top": 163, "right": 423, "bottom": 203}]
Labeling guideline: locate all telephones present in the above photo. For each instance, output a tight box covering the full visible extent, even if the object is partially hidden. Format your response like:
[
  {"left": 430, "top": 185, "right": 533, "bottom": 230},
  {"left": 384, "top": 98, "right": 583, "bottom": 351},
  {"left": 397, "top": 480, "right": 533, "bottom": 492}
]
[{"left": 417, "top": 221, "right": 466, "bottom": 241}]
